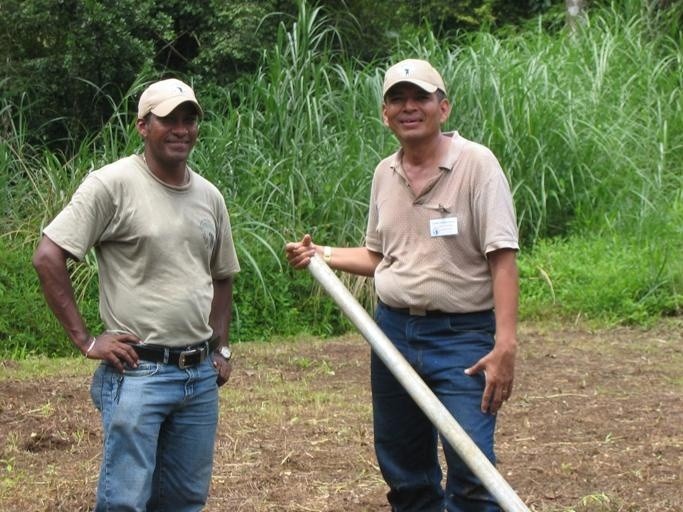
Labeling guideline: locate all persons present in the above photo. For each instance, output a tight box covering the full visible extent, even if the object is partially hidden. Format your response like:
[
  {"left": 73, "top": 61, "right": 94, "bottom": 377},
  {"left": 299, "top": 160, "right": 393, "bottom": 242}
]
[
  {"left": 31, "top": 76, "right": 242, "bottom": 511},
  {"left": 283, "top": 57, "right": 521, "bottom": 510}
]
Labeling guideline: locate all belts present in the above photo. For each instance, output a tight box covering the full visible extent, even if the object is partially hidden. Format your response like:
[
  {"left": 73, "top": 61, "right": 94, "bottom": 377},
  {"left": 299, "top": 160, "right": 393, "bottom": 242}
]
[
  {"left": 374, "top": 295, "right": 494, "bottom": 318},
  {"left": 120, "top": 334, "right": 222, "bottom": 369}
]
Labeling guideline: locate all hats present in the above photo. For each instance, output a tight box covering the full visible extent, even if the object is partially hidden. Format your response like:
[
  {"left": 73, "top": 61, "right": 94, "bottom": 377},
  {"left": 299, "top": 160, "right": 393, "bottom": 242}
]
[
  {"left": 381, "top": 56, "right": 450, "bottom": 101},
  {"left": 136, "top": 76, "right": 204, "bottom": 127}
]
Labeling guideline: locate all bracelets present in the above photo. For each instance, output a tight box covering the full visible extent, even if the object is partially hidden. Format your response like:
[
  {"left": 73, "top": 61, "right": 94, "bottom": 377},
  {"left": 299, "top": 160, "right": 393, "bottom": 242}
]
[
  {"left": 84, "top": 335, "right": 97, "bottom": 359},
  {"left": 321, "top": 245, "right": 331, "bottom": 268}
]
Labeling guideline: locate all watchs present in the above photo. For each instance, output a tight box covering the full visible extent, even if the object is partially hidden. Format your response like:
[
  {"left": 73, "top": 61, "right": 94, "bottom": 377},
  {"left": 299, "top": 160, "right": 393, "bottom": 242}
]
[{"left": 209, "top": 345, "right": 233, "bottom": 364}]
